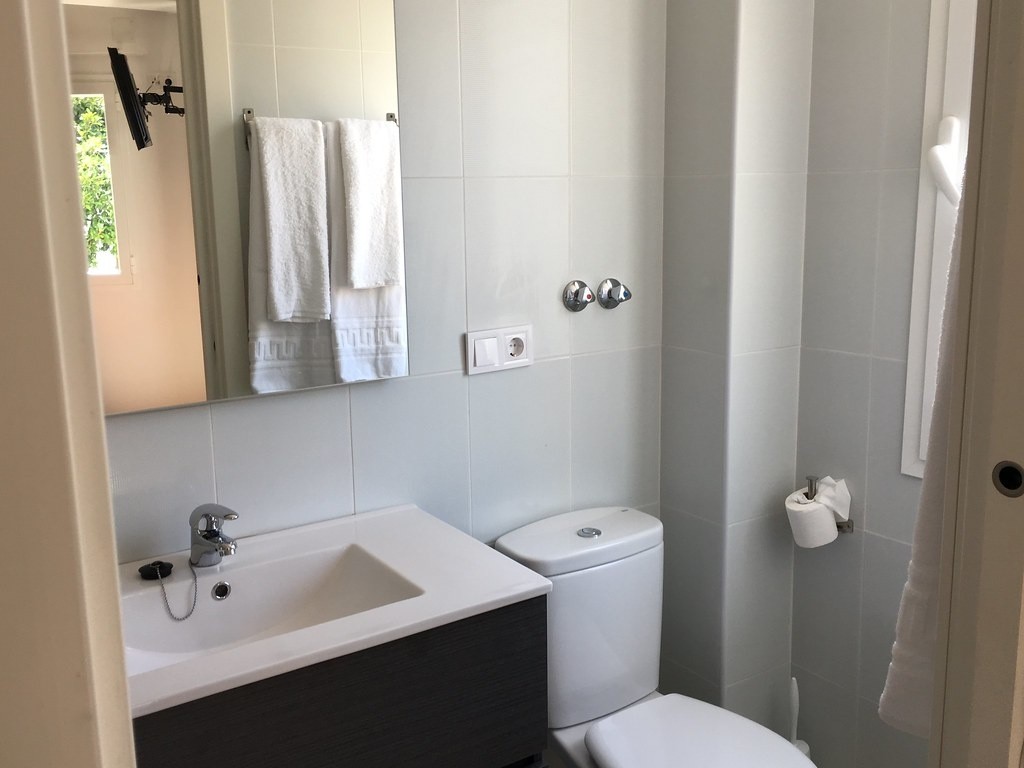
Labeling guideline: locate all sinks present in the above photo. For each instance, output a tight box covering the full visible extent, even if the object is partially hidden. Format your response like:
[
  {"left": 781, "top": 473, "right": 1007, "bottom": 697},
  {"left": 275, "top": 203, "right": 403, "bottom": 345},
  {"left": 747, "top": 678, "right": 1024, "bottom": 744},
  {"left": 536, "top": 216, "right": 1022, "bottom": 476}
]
[{"left": 120, "top": 536, "right": 427, "bottom": 678}]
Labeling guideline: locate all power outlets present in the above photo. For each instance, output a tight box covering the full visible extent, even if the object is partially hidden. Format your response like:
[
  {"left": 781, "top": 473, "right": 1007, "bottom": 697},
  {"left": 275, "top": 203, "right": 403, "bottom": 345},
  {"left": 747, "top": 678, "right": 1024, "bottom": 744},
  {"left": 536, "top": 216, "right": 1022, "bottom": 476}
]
[{"left": 466, "top": 324, "right": 533, "bottom": 376}]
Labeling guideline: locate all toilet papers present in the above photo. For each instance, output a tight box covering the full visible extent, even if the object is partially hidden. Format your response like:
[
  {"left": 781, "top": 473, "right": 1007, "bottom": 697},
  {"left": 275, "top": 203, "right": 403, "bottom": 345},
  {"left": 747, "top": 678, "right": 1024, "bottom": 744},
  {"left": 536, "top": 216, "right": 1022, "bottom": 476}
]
[{"left": 784, "top": 474, "right": 852, "bottom": 550}]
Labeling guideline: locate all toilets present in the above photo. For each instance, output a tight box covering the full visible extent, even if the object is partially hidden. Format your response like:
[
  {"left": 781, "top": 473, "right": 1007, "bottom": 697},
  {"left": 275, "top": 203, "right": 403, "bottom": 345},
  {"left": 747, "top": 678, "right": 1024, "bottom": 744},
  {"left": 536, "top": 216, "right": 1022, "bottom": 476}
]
[{"left": 494, "top": 505, "right": 819, "bottom": 768}]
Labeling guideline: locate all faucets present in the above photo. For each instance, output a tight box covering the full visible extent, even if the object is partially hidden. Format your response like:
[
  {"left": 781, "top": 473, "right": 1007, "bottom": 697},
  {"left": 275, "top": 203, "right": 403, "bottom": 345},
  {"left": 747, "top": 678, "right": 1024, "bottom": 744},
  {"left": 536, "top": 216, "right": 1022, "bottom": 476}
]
[{"left": 187, "top": 501, "right": 239, "bottom": 567}]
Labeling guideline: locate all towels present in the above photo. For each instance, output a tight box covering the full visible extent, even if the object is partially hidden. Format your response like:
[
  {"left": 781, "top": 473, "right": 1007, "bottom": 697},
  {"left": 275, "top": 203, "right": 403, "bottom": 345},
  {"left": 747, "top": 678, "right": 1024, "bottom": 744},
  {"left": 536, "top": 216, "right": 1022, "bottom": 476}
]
[
  {"left": 876, "top": 156, "right": 970, "bottom": 737},
  {"left": 244, "top": 117, "right": 327, "bottom": 396},
  {"left": 330, "top": 114, "right": 408, "bottom": 386}
]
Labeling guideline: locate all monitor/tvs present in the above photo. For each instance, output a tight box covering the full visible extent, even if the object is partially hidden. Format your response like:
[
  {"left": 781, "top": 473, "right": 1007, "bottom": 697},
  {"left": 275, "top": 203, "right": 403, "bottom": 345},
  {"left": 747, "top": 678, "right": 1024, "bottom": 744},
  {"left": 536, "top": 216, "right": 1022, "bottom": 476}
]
[{"left": 106, "top": 46, "right": 153, "bottom": 151}]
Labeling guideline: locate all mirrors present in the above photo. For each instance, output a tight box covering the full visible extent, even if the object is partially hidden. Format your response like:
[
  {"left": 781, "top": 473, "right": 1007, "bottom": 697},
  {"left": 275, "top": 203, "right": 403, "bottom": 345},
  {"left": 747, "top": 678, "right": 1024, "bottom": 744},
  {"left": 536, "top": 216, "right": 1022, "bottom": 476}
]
[{"left": 62, "top": 1, "right": 409, "bottom": 417}]
